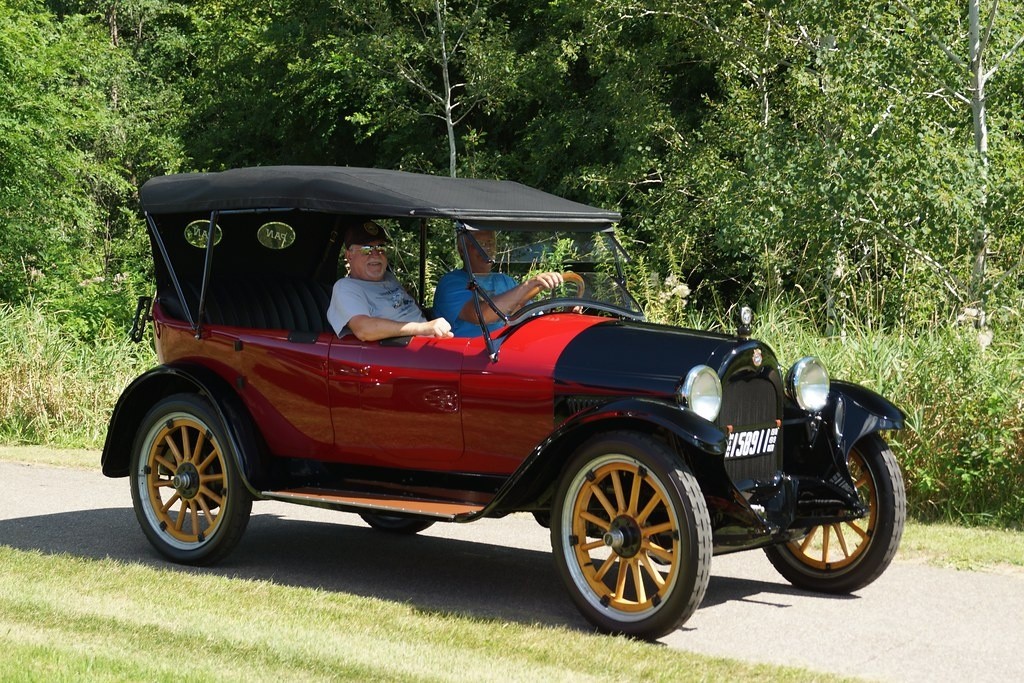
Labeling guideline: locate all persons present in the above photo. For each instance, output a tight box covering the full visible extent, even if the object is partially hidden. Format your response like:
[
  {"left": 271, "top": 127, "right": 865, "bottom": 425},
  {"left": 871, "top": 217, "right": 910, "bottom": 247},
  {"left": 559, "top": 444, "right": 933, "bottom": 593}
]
[
  {"left": 324, "top": 219, "right": 454, "bottom": 342},
  {"left": 432, "top": 226, "right": 583, "bottom": 338}
]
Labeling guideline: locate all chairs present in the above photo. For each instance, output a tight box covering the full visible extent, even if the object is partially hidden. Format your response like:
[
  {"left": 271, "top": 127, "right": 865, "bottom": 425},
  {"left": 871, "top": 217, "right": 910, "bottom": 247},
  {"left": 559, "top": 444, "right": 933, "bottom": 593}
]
[
  {"left": 424, "top": 306, "right": 434, "bottom": 321},
  {"left": 193, "top": 264, "right": 334, "bottom": 334}
]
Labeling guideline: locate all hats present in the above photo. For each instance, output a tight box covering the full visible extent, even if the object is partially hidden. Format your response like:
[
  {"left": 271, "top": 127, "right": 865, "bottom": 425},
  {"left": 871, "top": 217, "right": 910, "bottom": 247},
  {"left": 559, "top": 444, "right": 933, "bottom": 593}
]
[{"left": 345, "top": 220, "right": 393, "bottom": 249}]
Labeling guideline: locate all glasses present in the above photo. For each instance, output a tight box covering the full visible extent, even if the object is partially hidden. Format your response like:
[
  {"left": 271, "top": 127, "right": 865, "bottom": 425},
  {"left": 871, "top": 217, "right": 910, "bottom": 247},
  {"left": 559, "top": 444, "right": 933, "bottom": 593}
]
[
  {"left": 348, "top": 246, "right": 388, "bottom": 256},
  {"left": 478, "top": 240, "right": 496, "bottom": 247}
]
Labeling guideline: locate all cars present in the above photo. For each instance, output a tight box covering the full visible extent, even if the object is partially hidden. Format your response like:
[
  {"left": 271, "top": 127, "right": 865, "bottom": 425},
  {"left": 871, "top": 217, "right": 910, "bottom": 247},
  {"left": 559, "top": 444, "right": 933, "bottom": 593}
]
[{"left": 100, "top": 165, "right": 908, "bottom": 642}]
